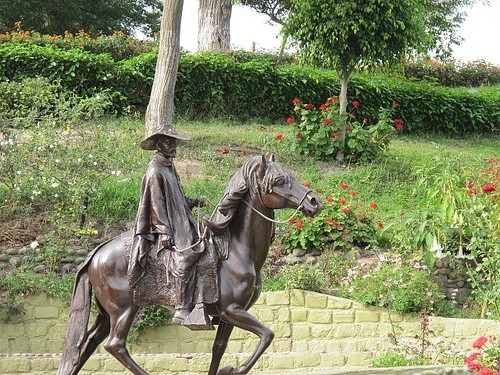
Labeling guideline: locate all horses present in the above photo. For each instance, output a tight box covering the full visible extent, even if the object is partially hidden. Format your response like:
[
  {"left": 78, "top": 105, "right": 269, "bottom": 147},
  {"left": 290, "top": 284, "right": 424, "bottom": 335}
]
[{"left": 56, "top": 152, "right": 322, "bottom": 375}]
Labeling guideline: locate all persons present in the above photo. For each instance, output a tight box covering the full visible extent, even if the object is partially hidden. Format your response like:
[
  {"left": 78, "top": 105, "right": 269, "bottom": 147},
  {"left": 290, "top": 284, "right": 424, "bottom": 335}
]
[{"left": 126, "top": 123, "right": 208, "bottom": 325}]
